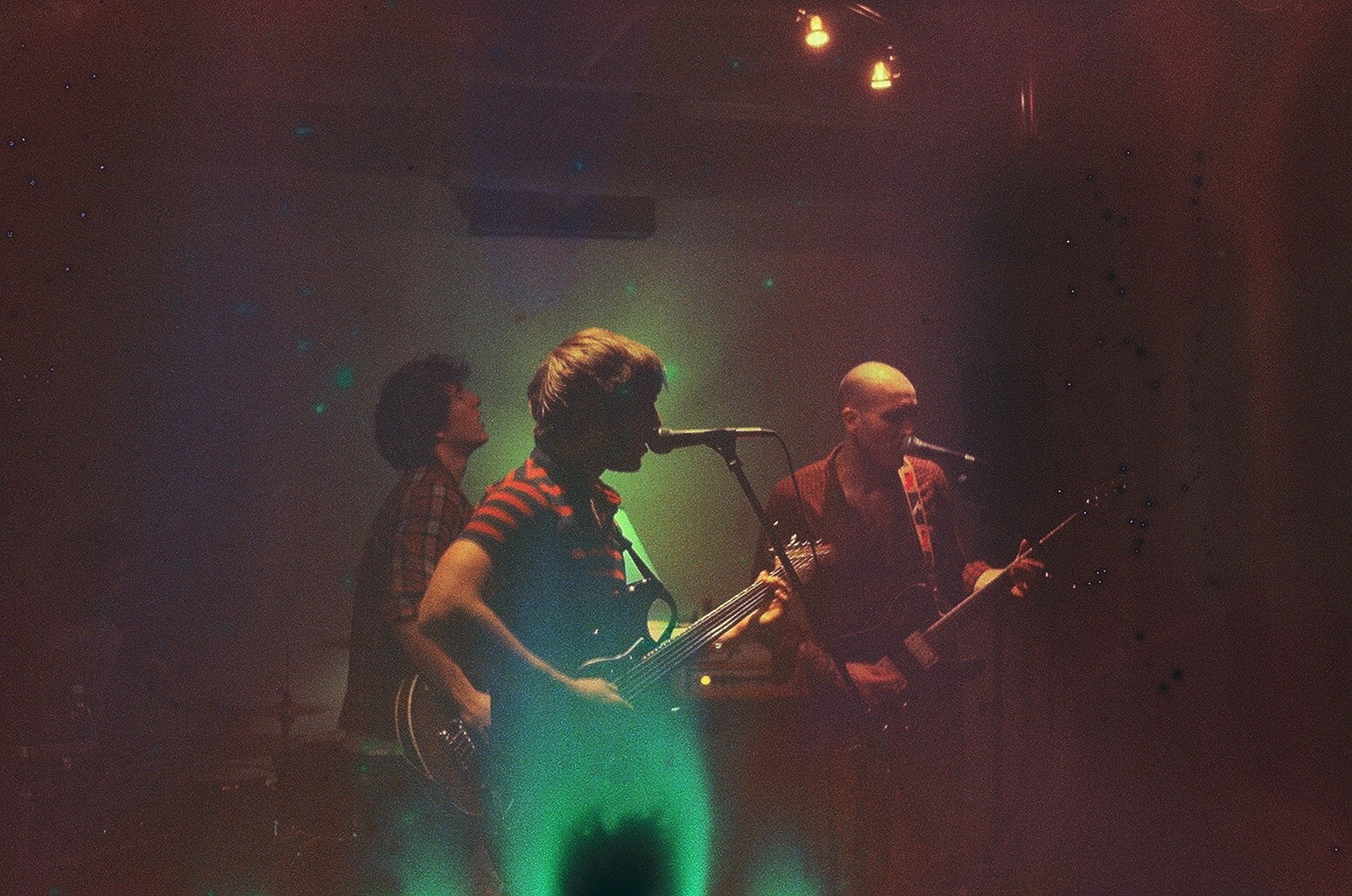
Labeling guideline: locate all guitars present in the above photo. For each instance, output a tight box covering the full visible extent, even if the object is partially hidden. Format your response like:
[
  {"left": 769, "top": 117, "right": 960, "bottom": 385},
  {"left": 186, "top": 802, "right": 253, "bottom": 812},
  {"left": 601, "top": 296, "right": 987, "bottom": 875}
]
[
  {"left": 391, "top": 667, "right": 519, "bottom": 818},
  {"left": 563, "top": 532, "right": 835, "bottom": 755},
  {"left": 817, "top": 466, "right": 1146, "bottom": 755}
]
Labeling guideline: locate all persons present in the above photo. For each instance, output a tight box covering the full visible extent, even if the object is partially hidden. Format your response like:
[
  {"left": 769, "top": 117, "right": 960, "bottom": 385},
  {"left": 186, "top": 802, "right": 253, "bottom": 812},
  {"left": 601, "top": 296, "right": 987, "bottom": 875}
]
[
  {"left": 342, "top": 357, "right": 498, "bottom": 895},
  {"left": 415, "top": 330, "right": 790, "bottom": 885},
  {"left": 753, "top": 361, "right": 1047, "bottom": 896}
]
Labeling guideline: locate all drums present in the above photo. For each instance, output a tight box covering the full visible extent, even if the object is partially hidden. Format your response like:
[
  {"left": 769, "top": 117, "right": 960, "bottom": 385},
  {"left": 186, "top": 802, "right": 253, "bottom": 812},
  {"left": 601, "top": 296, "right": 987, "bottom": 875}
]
[{"left": 179, "top": 763, "right": 283, "bottom": 859}]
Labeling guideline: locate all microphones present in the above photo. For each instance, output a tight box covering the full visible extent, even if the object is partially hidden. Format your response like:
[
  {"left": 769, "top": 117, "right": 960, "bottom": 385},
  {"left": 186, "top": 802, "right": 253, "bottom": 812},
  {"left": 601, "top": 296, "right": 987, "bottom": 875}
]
[
  {"left": 901, "top": 436, "right": 987, "bottom": 464},
  {"left": 647, "top": 427, "right": 776, "bottom": 454}
]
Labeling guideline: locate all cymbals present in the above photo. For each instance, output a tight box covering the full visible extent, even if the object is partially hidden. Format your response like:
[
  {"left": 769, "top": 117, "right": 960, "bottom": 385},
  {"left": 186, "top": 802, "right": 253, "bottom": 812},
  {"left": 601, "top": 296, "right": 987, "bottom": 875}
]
[{"left": 225, "top": 698, "right": 339, "bottom": 721}]
